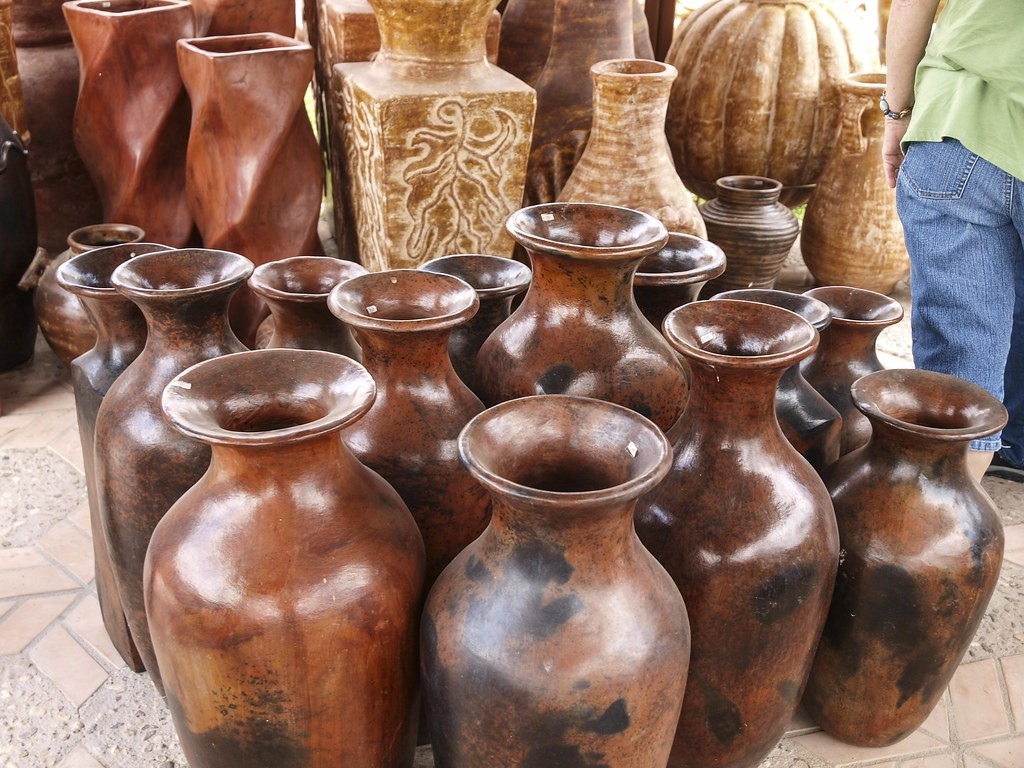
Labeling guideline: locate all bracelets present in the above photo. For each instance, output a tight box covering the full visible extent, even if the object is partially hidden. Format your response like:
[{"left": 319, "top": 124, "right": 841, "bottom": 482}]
[{"left": 880, "top": 89, "right": 913, "bottom": 120}]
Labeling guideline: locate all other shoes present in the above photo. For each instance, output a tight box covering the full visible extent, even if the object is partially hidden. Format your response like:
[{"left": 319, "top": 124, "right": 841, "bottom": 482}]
[{"left": 987, "top": 452, "right": 1024, "bottom": 481}]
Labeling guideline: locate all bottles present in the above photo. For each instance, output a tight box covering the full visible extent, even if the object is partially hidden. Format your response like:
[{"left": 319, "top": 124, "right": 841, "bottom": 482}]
[{"left": 1, "top": 0, "right": 1009, "bottom": 768}]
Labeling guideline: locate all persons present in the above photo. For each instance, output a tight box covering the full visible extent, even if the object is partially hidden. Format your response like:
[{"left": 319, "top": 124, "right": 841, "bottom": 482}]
[{"left": 882, "top": 0, "right": 1024, "bottom": 482}]
